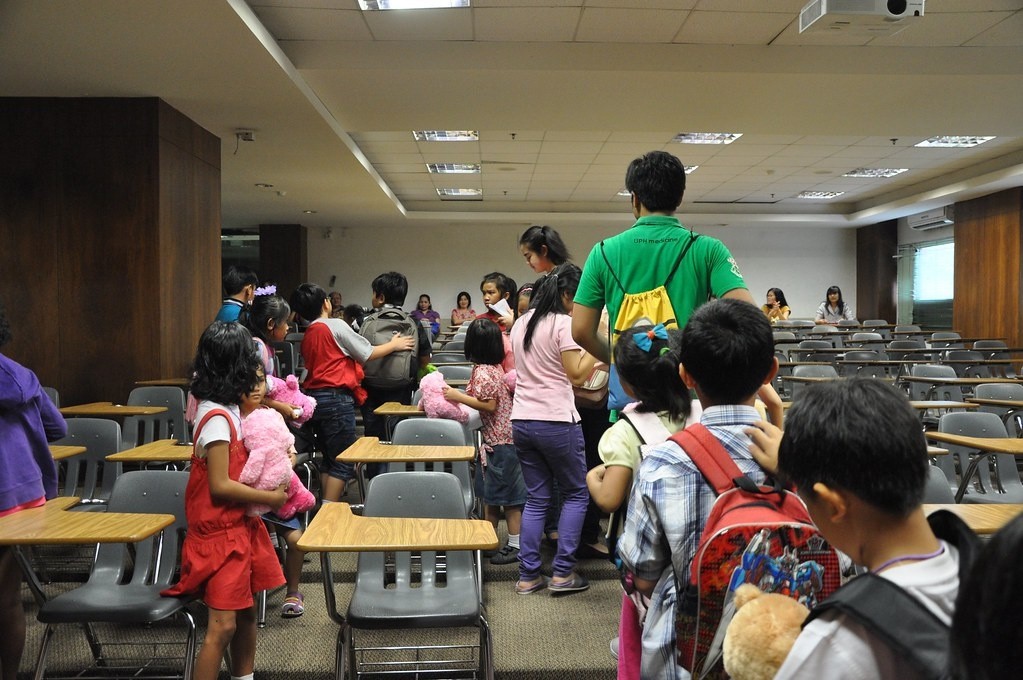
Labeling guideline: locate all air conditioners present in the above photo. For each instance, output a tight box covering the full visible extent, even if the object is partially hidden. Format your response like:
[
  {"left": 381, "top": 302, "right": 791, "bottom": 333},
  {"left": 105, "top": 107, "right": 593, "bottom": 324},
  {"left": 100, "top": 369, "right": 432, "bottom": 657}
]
[{"left": 906, "top": 203, "right": 954, "bottom": 231}]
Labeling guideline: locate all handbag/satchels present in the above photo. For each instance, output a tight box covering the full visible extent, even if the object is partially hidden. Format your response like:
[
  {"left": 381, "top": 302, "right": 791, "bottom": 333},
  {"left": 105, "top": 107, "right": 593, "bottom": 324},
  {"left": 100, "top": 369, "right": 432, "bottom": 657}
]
[{"left": 571, "top": 361, "right": 610, "bottom": 411}]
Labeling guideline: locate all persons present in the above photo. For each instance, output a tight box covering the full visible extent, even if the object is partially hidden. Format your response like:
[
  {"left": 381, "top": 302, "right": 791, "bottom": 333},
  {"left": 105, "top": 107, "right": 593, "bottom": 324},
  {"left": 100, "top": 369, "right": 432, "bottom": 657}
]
[
  {"left": 520, "top": 225, "right": 573, "bottom": 273},
  {"left": 517, "top": 283, "right": 534, "bottom": 322},
  {"left": 162, "top": 321, "right": 289, "bottom": 680},
  {"left": 742, "top": 375, "right": 962, "bottom": 680},
  {"left": 410, "top": 294, "right": 440, "bottom": 341},
  {"left": 443, "top": 317, "right": 526, "bottom": 564},
  {"left": 293, "top": 282, "right": 416, "bottom": 504},
  {"left": 360, "top": 272, "right": 433, "bottom": 441},
  {"left": 215, "top": 266, "right": 258, "bottom": 328},
  {"left": 1, "top": 355, "right": 68, "bottom": 680},
  {"left": 451, "top": 292, "right": 475, "bottom": 330},
  {"left": 571, "top": 149, "right": 1023, "bottom": 680},
  {"left": 475, "top": 272, "right": 517, "bottom": 331},
  {"left": 509, "top": 263, "right": 597, "bottom": 593},
  {"left": 185, "top": 284, "right": 289, "bottom": 433}
]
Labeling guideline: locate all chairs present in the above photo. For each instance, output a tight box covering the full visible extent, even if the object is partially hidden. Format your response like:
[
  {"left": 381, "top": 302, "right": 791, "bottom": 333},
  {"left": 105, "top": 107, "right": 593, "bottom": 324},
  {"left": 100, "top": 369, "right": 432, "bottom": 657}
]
[{"left": 0, "top": 320, "right": 1023, "bottom": 680}]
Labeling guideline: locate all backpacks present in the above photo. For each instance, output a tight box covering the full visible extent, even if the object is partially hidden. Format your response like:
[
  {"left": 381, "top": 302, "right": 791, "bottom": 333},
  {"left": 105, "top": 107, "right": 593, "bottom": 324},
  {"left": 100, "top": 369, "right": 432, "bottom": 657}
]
[
  {"left": 619, "top": 401, "right": 703, "bottom": 630},
  {"left": 665, "top": 423, "right": 842, "bottom": 680},
  {"left": 600, "top": 225, "right": 702, "bottom": 410},
  {"left": 359, "top": 308, "right": 418, "bottom": 386}
]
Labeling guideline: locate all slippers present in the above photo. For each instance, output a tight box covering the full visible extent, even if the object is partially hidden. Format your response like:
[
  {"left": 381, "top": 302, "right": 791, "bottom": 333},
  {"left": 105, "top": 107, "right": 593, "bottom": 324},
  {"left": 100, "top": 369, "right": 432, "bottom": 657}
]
[
  {"left": 515, "top": 574, "right": 551, "bottom": 595},
  {"left": 548, "top": 572, "right": 590, "bottom": 591}
]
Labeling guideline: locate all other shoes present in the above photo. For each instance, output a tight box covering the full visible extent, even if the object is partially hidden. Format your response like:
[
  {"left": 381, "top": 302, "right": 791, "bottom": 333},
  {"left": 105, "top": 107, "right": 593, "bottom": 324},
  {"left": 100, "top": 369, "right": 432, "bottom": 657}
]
[
  {"left": 282, "top": 593, "right": 305, "bottom": 616},
  {"left": 490, "top": 545, "right": 520, "bottom": 565},
  {"left": 546, "top": 535, "right": 557, "bottom": 547},
  {"left": 577, "top": 544, "right": 609, "bottom": 559},
  {"left": 610, "top": 637, "right": 619, "bottom": 660},
  {"left": 483, "top": 550, "right": 497, "bottom": 557}
]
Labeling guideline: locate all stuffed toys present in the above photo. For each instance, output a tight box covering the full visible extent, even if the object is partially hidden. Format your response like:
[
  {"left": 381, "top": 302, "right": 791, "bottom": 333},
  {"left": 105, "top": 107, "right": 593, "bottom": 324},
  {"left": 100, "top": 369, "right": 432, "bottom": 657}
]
[
  {"left": 418, "top": 371, "right": 469, "bottom": 424},
  {"left": 240, "top": 407, "right": 316, "bottom": 518},
  {"left": 723, "top": 582, "right": 812, "bottom": 680},
  {"left": 265, "top": 373, "right": 317, "bottom": 430}
]
